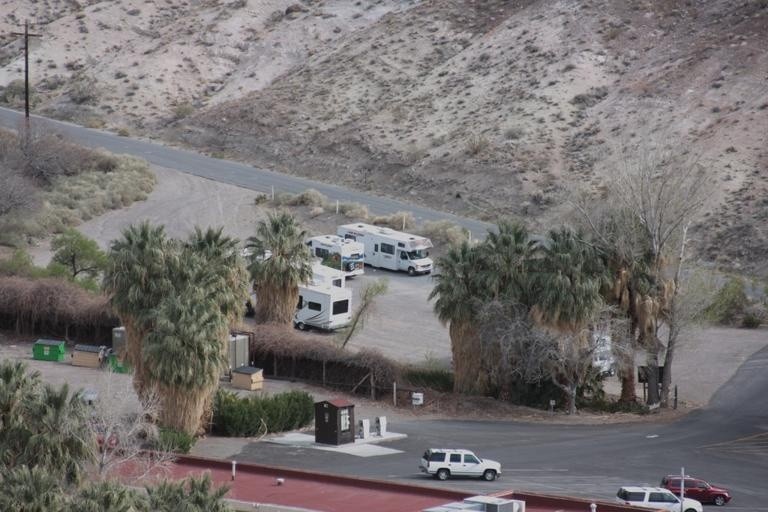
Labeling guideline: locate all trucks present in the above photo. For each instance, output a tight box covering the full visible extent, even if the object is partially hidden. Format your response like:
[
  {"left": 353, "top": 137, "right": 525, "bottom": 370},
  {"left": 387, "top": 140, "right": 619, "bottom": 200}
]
[{"left": 237, "top": 221, "right": 435, "bottom": 333}]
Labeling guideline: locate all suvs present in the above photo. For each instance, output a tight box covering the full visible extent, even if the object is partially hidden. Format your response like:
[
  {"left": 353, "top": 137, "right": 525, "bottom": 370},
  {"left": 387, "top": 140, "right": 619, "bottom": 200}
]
[
  {"left": 661, "top": 473, "right": 733, "bottom": 507},
  {"left": 416, "top": 446, "right": 503, "bottom": 481},
  {"left": 617, "top": 485, "right": 704, "bottom": 511}
]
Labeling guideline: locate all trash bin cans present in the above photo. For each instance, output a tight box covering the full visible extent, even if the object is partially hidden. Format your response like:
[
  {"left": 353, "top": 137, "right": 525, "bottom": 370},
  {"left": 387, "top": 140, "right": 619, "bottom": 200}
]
[
  {"left": 33, "top": 339, "right": 64, "bottom": 361},
  {"left": 109, "top": 352, "right": 135, "bottom": 373}
]
